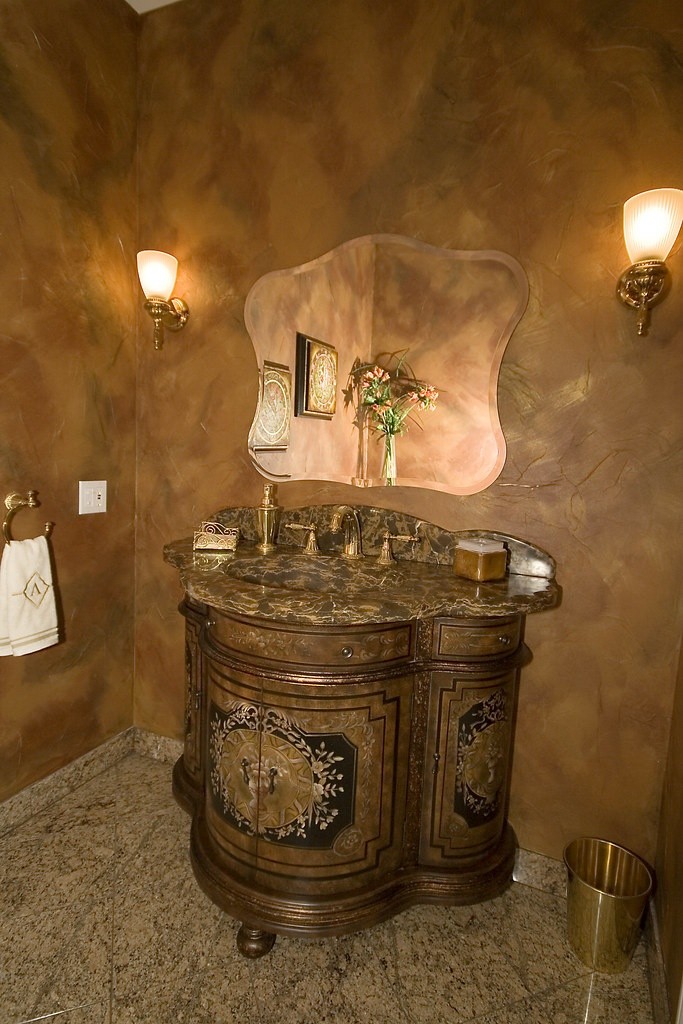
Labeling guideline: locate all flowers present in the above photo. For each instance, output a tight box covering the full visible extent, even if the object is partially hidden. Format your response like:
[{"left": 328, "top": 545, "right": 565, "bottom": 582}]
[{"left": 349, "top": 348, "right": 447, "bottom": 486}]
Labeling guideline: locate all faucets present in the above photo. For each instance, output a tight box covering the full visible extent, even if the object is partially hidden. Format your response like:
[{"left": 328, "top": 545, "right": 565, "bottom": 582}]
[{"left": 330, "top": 505, "right": 366, "bottom": 561}]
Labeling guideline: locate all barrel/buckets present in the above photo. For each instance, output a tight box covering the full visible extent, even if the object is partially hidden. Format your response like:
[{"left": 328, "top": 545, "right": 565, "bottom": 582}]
[{"left": 563, "top": 837, "right": 653, "bottom": 974}]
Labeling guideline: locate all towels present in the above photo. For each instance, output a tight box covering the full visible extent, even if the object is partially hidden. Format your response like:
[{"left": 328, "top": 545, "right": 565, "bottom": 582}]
[{"left": 1, "top": 534, "right": 61, "bottom": 658}]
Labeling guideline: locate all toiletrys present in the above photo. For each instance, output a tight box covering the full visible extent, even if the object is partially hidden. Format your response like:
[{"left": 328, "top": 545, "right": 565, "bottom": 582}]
[{"left": 256, "top": 483, "right": 279, "bottom": 553}]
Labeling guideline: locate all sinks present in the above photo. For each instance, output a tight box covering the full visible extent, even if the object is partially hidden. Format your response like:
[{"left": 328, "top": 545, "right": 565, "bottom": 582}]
[{"left": 220, "top": 553, "right": 407, "bottom": 595}]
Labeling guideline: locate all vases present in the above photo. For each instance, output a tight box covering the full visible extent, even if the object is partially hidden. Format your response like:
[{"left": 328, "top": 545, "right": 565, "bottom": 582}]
[{"left": 379, "top": 435, "right": 397, "bottom": 486}]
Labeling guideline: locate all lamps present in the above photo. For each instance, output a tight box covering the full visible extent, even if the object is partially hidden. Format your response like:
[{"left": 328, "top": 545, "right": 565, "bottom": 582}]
[
  {"left": 136, "top": 249, "right": 189, "bottom": 351},
  {"left": 616, "top": 187, "right": 683, "bottom": 335}
]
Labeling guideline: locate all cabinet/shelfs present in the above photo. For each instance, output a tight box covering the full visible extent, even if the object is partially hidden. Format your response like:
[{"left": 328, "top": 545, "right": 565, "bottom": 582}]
[{"left": 171, "top": 594, "right": 533, "bottom": 957}]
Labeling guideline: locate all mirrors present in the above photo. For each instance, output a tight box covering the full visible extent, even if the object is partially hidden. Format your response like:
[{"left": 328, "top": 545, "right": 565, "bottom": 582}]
[{"left": 244, "top": 233, "right": 529, "bottom": 495}]
